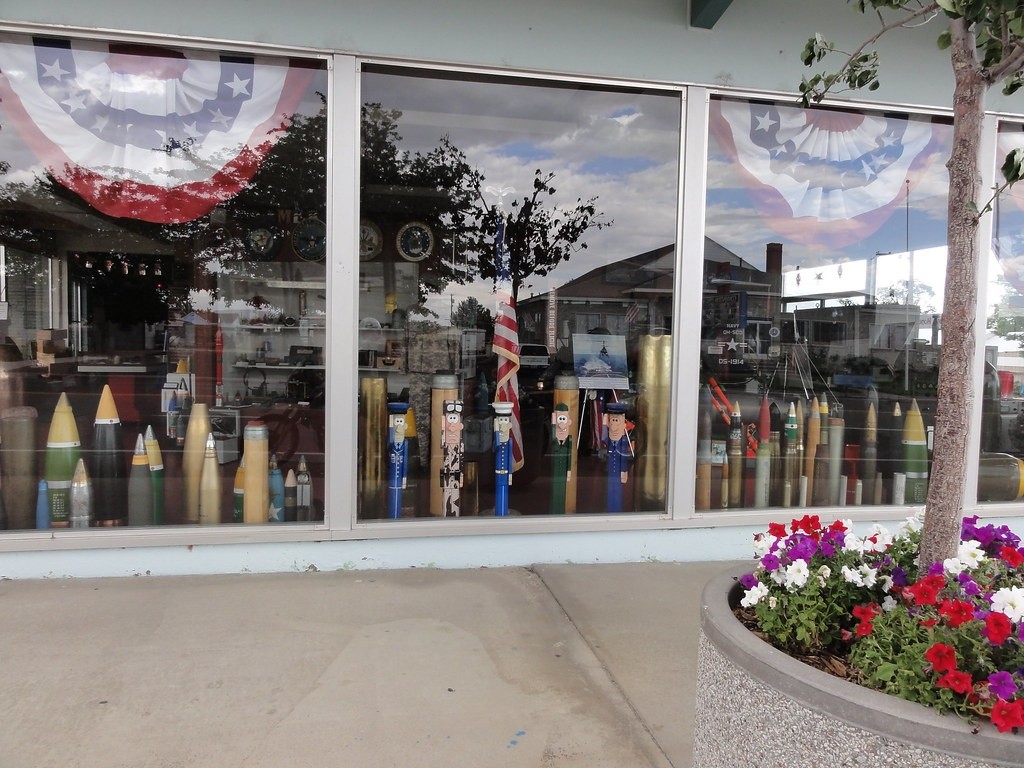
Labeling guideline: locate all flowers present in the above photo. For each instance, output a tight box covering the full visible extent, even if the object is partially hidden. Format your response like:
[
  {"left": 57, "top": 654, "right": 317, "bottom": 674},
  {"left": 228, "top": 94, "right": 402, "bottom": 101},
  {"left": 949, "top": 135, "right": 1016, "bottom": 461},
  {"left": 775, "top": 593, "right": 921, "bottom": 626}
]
[{"left": 739, "top": 504, "right": 1024, "bottom": 736}]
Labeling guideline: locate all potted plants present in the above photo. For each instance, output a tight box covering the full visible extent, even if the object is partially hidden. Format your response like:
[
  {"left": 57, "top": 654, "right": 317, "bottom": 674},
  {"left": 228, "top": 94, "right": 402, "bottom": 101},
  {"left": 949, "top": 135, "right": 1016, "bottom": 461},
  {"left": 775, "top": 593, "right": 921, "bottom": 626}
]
[{"left": 695, "top": 0, "right": 1024, "bottom": 768}]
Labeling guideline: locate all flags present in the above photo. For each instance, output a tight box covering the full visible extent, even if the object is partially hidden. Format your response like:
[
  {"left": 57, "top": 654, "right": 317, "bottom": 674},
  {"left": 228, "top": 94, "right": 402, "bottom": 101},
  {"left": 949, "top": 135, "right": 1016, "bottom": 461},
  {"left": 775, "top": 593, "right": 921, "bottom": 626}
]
[{"left": 491, "top": 208, "right": 527, "bottom": 472}]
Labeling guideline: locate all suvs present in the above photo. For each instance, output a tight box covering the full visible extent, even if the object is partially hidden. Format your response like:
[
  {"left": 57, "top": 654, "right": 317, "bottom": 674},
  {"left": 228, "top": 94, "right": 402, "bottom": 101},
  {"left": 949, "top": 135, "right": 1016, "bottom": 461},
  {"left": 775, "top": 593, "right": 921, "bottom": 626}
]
[{"left": 517, "top": 343, "right": 550, "bottom": 373}]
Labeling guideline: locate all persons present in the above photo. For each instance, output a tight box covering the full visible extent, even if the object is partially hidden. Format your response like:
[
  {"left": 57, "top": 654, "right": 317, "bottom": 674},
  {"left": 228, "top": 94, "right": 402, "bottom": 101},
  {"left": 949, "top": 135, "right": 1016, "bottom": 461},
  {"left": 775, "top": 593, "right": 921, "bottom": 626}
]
[
  {"left": 492, "top": 402, "right": 514, "bottom": 516},
  {"left": 440, "top": 400, "right": 464, "bottom": 518},
  {"left": 549, "top": 403, "right": 572, "bottom": 513},
  {"left": 602, "top": 403, "right": 629, "bottom": 511},
  {"left": 624, "top": 292, "right": 639, "bottom": 323},
  {"left": 388, "top": 402, "right": 410, "bottom": 519}
]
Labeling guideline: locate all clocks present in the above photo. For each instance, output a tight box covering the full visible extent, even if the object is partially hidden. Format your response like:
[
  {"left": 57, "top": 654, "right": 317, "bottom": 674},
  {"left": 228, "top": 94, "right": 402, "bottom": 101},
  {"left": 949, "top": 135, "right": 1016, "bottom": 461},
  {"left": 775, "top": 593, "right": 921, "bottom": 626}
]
[{"left": 360, "top": 218, "right": 383, "bottom": 262}]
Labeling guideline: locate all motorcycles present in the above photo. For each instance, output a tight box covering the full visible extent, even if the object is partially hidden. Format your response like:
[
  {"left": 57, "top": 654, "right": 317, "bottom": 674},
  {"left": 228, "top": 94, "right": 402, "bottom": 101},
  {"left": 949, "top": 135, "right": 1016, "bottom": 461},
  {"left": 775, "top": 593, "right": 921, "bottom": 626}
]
[
  {"left": 238, "top": 355, "right": 325, "bottom": 468},
  {"left": 518, "top": 376, "right": 649, "bottom": 464}
]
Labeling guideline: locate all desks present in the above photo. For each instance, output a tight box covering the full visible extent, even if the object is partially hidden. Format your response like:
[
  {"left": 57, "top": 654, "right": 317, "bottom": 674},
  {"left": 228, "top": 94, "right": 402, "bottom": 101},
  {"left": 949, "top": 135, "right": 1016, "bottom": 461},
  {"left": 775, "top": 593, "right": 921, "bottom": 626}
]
[{"left": 77, "top": 363, "right": 166, "bottom": 424}]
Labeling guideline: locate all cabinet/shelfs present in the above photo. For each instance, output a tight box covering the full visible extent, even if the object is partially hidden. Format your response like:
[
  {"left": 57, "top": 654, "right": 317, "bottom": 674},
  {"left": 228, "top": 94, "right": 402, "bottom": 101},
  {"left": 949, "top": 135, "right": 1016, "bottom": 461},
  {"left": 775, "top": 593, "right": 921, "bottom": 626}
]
[{"left": 229, "top": 324, "right": 406, "bottom": 373}]
